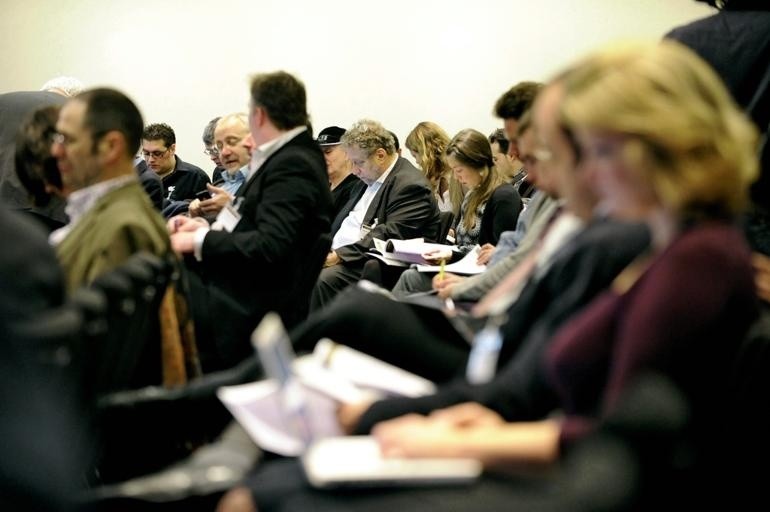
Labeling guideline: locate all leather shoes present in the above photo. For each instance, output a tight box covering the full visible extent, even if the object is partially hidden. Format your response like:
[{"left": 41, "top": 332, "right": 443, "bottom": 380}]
[{"left": 97, "top": 386, "right": 219, "bottom": 441}]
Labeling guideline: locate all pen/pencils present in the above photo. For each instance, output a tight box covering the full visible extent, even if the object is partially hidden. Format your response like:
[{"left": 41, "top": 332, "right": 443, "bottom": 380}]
[{"left": 438, "top": 260, "right": 446, "bottom": 281}]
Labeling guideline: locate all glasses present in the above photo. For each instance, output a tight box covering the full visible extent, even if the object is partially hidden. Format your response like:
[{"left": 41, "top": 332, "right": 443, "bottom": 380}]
[
  {"left": 204, "top": 147, "right": 219, "bottom": 156},
  {"left": 50, "top": 127, "right": 104, "bottom": 145},
  {"left": 141, "top": 149, "right": 170, "bottom": 160}
]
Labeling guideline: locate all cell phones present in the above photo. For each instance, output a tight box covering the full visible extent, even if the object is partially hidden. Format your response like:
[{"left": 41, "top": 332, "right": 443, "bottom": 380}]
[{"left": 195, "top": 190, "right": 211, "bottom": 201}]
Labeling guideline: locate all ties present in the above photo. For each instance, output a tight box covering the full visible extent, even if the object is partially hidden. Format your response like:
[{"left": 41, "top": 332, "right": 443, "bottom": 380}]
[{"left": 469, "top": 206, "right": 564, "bottom": 320}]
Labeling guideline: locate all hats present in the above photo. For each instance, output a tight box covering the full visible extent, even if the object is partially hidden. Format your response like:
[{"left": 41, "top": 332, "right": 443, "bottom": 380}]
[
  {"left": 530, "top": 140, "right": 575, "bottom": 164},
  {"left": 314, "top": 126, "right": 346, "bottom": 146}
]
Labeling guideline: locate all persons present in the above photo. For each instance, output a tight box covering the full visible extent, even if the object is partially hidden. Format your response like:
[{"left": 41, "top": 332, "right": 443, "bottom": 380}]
[
  {"left": 356, "top": 109, "right": 576, "bottom": 321},
  {"left": 233, "top": 113, "right": 313, "bottom": 198},
  {"left": 307, "top": 119, "right": 455, "bottom": 320},
  {"left": 88, "top": 108, "right": 564, "bottom": 445},
  {"left": 661, "top": 0, "right": 770, "bottom": 305},
  {"left": 405, "top": 121, "right": 473, "bottom": 230},
  {"left": 139, "top": 122, "right": 212, "bottom": 212},
  {"left": 201, "top": 117, "right": 225, "bottom": 183},
  {"left": 390, "top": 128, "right": 524, "bottom": 296},
  {"left": 488, "top": 127, "right": 542, "bottom": 208},
  {"left": 187, "top": 111, "right": 253, "bottom": 226},
  {"left": 316, "top": 127, "right": 363, "bottom": 224},
  {"left": 0, "top": 92, "right": 116, "bottom": 512},
  {"left": 222, "top": 34, "right": 761, "bottom": 510},
  {"left": 334, "top": 67, "right": 665, "bottom": 436}
]
